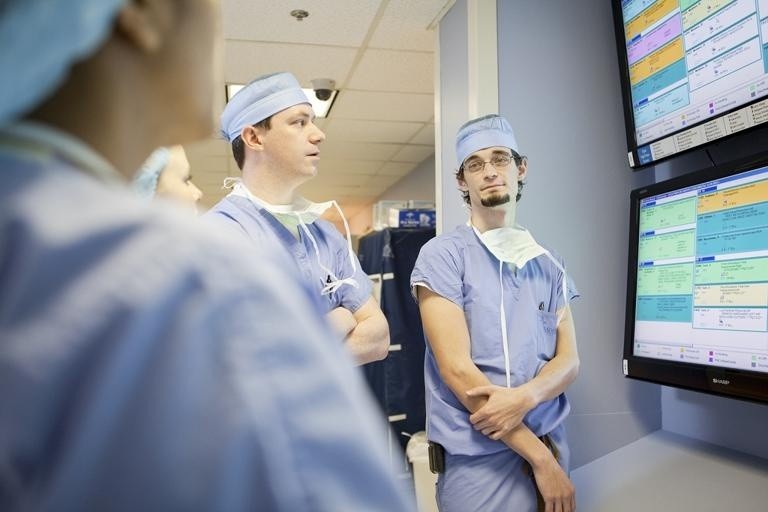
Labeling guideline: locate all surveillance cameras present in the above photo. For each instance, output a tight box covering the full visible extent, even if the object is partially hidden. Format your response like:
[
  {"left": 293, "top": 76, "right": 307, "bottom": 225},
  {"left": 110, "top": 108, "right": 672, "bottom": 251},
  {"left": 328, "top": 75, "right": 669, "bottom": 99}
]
[{"left": 312, "top": 80, "right": 336, "bottom": 101}]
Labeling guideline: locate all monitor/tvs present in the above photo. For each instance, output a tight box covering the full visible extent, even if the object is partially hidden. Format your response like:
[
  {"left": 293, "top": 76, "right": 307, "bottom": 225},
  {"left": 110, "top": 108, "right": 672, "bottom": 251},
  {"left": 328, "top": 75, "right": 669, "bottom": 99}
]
[
  {"left": 610, "top": 0, "right": 768, "bottom": 171},
  {"left": 621, "top": 153, "right": 768, "bottom": 406}
]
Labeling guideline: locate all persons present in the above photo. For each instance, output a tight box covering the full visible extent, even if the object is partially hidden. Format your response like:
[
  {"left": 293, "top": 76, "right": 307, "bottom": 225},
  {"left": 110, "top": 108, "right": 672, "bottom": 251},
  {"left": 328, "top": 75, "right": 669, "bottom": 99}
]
[
  {"left": 135, "top": 143, "right": 205, "bottom": 215},
  {"left": 203, "top": 72, "right": 390, "bottom": 365},
  {"left": 0, "top": 0, "right": 418, "bottom": 512},
  {"left": 408, "top": 118, "right": 582, "bottom": 512}
]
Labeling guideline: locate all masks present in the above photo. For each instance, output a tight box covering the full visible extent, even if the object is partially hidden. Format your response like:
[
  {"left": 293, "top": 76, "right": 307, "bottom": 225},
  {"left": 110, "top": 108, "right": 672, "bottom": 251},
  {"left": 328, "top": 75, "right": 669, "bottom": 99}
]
[
  {"left": 273, "top": 198, "right": 334, "bottom": 229},
  {"left": 477, "top": 224, "right": 546, "bottom": 269}
]
